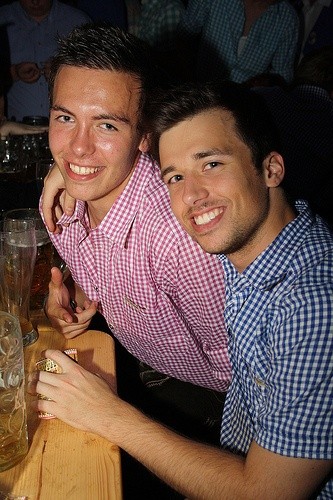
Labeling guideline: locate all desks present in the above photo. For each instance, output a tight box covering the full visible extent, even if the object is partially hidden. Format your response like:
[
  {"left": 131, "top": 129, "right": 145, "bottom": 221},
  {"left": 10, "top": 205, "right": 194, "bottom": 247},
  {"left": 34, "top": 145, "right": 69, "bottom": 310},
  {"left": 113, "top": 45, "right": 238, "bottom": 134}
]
[{"left": 1, "top": 324, "right": 123, "bottom": 500}]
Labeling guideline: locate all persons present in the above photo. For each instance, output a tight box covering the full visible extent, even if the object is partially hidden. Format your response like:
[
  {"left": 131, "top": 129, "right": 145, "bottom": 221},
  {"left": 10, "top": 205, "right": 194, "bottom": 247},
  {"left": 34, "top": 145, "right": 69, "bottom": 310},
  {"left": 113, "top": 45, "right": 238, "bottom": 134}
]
[
  {"left": 25, "top": 85, "right": 333, "bottom": 500},
  {"left": 37, "top": 26, "right": 233, "bottom": 397},
  {"left": 0, "top": 0, "right": 332, "bottom": 163}
]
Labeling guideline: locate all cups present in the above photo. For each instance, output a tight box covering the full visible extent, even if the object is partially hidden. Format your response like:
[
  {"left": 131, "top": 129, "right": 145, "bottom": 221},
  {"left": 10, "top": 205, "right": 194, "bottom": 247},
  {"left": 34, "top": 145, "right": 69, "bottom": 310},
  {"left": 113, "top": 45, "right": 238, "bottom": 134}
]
[
  {"left": 36, "top": 140, "right": 53, "bottom": 182},
  {"left": 0, "top": 134, "right": 34, "bottom": 174},
  {"left": 0, "top": 219, "right": 39, "bottom": 347},
  {"left": 0, "top": 311, "right": 29, "bottom": 473},
  {"left": 2, "top": 208, "right": 53, "bottom": 310}
]
